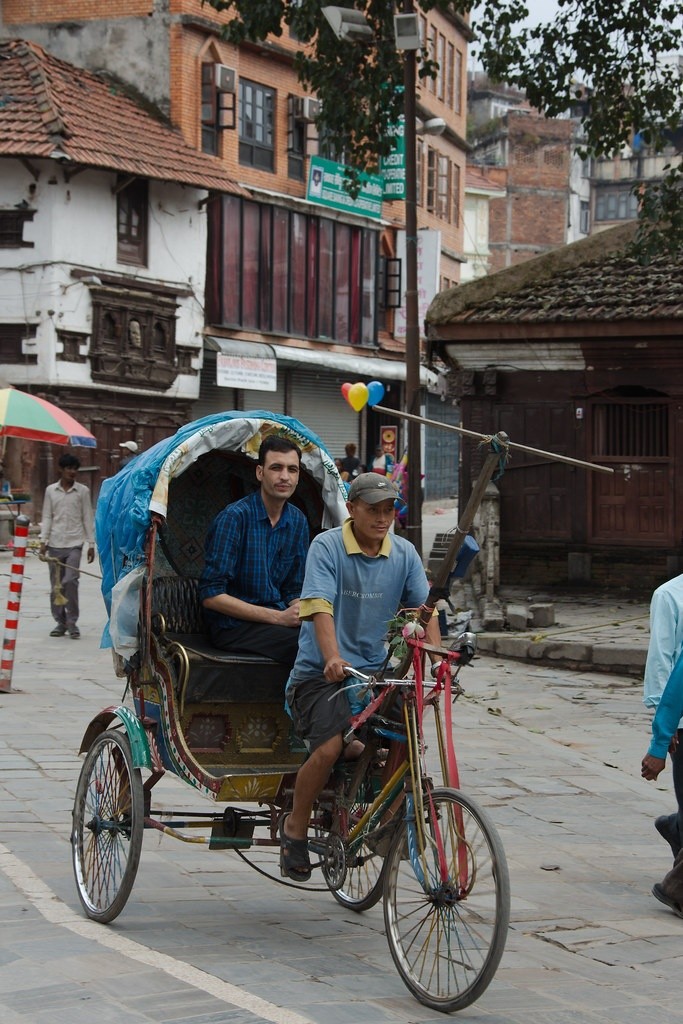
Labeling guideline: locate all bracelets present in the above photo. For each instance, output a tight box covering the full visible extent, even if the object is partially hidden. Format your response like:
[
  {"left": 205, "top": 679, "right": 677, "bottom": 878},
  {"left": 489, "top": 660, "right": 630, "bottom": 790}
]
[{"left": 431, "top": 661, "right": 442, "bottom": 677}]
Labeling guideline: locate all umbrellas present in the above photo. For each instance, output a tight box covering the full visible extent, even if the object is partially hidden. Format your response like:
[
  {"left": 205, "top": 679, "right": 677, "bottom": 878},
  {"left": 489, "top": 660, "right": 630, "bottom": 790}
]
[{"left": 0, "top": 388, "right": 97, "bottom": 453}]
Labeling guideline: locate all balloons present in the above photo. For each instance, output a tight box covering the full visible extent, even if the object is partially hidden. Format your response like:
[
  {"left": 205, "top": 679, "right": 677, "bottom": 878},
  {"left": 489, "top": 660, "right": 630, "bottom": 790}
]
[{"left": 339, "top": 381, "right": 384, "bottom": 413}]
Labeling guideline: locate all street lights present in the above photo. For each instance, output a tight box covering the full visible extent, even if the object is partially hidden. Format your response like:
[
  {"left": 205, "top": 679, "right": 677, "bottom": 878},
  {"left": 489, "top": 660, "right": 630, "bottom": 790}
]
[{"left": 319, "top": 3, "right": 448, "bottom": 559}]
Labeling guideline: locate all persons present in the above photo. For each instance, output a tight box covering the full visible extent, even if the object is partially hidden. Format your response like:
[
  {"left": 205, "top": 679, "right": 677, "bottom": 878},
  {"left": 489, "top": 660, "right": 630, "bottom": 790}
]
[
  {"left": 192, "top": 436, "right": 397, "bottom": 764},
  {"left": 38, "top": 455, "right": 94, "bottom": 639},
  {"left": 284, "top": 483, "right": 450, "bottom": 881},
  {"left": 641, "top": 574, "right": 683, "bottom": 919},
  {"left": 119, "top": 440, "right": 138, "bottom": 472},
  {"left": 130, "top": 321, "right": 141, "bottom": 347},
  {"left": 333, "top": 443, "right": 397, "bottom": 483}
]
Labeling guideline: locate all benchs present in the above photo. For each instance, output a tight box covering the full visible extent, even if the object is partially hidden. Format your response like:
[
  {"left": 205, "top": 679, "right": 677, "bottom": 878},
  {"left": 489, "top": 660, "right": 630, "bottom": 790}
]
[{"left": 152, "top": 575, "right": 279, "bottom": 704}]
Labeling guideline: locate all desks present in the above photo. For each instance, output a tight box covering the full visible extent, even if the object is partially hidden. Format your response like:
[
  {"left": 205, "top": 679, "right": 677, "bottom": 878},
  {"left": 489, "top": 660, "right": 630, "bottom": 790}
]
[{"left": 0, "top": 500, "right": 33, "bottom": 518}]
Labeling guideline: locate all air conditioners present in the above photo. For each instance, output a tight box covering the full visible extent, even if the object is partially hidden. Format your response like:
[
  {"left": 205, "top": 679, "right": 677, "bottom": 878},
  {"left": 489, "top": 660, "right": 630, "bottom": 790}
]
[
  {"left": 208, "top": 64, "right": 237, "bottom": 95},
  {"left": 293, "top": 97, "right": 318, "bottom": 124}
]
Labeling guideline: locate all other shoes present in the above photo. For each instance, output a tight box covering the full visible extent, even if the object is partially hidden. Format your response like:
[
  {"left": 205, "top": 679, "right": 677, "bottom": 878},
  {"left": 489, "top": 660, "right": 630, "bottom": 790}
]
[
  {"left": 69, "top": 626, "right": 80, "bottom": 637},
  {"left": 652, "top": 883, "right": 683, "bottom": 922},
  {"left": 50, "top": 625, "right": 66, "bottom": 636},
  {"left": 654, "top": 815, "right": 681, "bottom": 860}
]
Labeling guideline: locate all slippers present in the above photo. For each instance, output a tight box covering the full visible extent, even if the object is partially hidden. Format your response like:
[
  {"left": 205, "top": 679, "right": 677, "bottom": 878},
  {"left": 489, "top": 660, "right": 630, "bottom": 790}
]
[{"left": 278, "top": 812, "right": 313, "bottom": 882}]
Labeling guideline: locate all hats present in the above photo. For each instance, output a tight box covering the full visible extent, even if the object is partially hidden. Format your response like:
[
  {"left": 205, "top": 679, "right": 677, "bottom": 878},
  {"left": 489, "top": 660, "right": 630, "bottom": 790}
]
[
  {"left": 119, "top": 441, "right": 138, "bottom": 453},
  {"left": 348, "top": 472, "right": 408, "bottom": 506}
]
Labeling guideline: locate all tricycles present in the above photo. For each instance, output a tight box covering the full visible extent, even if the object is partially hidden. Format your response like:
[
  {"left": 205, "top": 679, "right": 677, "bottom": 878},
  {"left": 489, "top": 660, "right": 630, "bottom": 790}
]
[{"left": 69, "top": 412, "right": 512, "bottom": 1012}]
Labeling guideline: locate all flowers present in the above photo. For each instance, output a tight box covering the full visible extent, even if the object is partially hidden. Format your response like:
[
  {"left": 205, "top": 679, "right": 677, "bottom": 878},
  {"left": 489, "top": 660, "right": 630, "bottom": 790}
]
[{"left": 381, "top": 610, "right": 426, "bottom": 658}]
